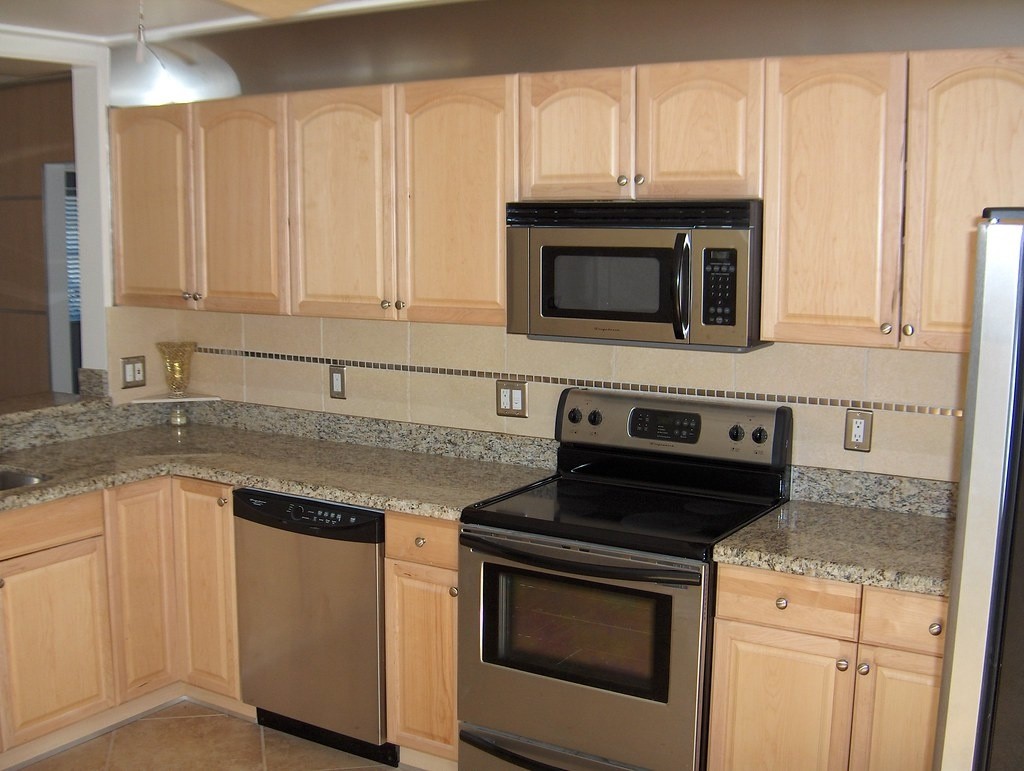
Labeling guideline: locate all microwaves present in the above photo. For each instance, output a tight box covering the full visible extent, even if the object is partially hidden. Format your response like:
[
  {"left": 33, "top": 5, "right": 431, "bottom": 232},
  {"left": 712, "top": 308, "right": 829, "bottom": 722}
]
[{"left": 505, "top": 200, "right": 775, "bottom": 354}]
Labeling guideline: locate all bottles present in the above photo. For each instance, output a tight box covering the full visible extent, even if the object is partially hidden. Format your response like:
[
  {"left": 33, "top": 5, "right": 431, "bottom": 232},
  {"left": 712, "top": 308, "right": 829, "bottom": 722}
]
[{"left": 171, "top": 402, "right": 187, "bottom": 427}]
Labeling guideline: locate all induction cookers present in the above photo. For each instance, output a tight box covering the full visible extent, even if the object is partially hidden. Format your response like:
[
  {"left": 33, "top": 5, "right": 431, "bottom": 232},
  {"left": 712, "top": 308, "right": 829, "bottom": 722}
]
[{"left": 460, "top": 387, "right": 793, "bottom": 562}]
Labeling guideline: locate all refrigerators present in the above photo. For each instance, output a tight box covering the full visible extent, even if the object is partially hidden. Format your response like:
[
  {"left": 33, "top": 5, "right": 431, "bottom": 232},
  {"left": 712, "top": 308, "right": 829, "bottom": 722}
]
[{"left": 932, "top": 206, "right": 1024, "bottom": 771}]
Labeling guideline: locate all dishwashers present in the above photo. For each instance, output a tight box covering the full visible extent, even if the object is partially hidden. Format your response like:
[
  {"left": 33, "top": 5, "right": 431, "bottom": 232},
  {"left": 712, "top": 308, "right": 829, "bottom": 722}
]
[{"left": 232, "top": 488, "right": 400, "bottom": 769}]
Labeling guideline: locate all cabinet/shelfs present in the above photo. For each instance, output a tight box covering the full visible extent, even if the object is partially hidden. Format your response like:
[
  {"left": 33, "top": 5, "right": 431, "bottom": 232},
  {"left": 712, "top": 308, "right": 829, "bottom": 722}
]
[
  {"left": 760, "top": 44, "right": 1024, "bottom": 354},
  {"left": 172, "top": 473, "right": 241, "bottom": 701},
  {"left": 706, "top": 560, "right": 950, "bottom": 771},
  {"left": 383, "top": 508, "right": 459, "bottom": 762},
  {"left": 290, "top": 71, "right": 519, "bottom": 325},
  {"left": 109, "top": 92, "right": 291, "bottom": 316},
  {"left": 0, "top": 488, "right": 115, "bottom": 751},
  {"left": 520, "top": 57, "right": 766, "bottom": 199}
]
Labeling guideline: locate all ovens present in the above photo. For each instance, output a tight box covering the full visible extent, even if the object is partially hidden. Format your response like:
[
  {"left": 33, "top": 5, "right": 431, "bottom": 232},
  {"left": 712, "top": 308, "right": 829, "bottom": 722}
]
[{"left": 457, "top": 535, "right": 715, "bottom": 771}]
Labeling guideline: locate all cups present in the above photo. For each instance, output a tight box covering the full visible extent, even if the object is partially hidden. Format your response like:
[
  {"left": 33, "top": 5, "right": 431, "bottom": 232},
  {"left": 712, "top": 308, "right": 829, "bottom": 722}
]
[{"left": 157, "top": 342, "right": 196, "bottom": 398}]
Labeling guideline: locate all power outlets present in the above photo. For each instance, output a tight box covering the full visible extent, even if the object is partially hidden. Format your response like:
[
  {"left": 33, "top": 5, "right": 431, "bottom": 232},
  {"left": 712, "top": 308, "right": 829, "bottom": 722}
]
[
  {"left": 120, "top": 355, "right": 145, "bottom": 389},
  {"left": 844, "top": 408, "right": 873, "bottom": 453},
  {"left": 496, "top": 379, "right": 528, "bottom": 418},
  {"left": 329, "top": 364, "right": 347, "bottom": 399}
]
